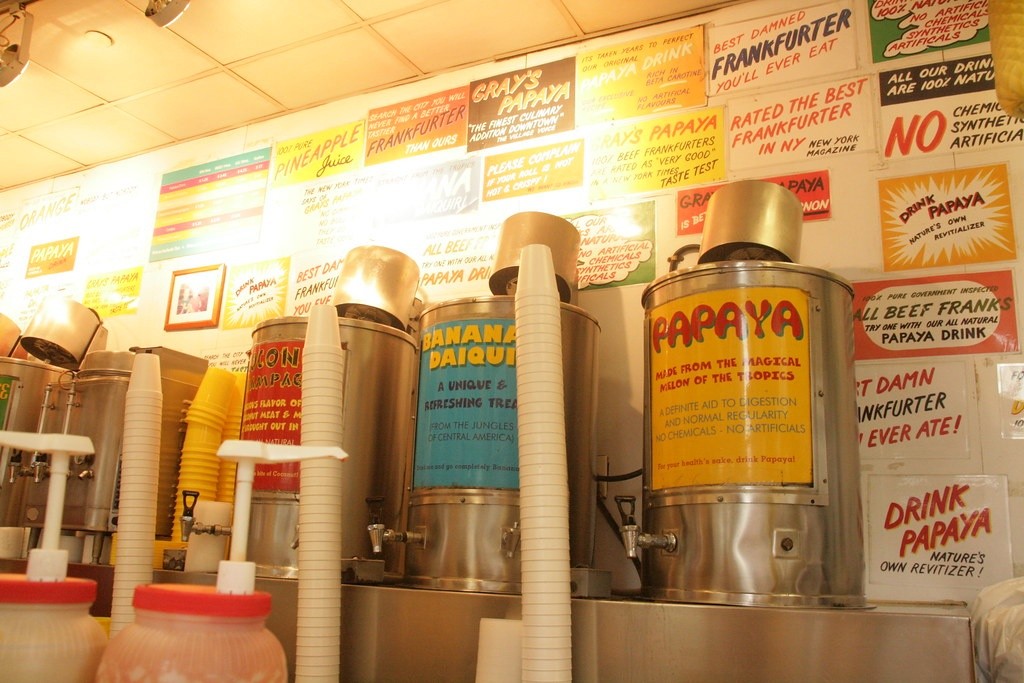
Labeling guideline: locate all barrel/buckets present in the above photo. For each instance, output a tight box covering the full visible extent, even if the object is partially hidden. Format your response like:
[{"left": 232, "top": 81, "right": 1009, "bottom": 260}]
[
  {"left": 234, "top": 315, "right": 419, "bottom": 579},
  {"left": 1, "top": 356, "right": 76, "bottom": 527},
  {"left": 640, "top": 263, "right": 867, "bottom": 610},
  {"left": 400, "top": 296, "right": 601, "bottom": 594}
]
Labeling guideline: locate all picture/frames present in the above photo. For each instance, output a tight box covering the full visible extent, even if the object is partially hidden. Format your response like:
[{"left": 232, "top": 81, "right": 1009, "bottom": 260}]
[{"left": 162, "top": 263, "right": 227, "bottom": 332}]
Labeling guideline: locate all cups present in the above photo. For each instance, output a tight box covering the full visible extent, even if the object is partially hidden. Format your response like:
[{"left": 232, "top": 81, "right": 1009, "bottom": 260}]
[
  {"left": 514, "top": 244, "right": 572, "bottom": 683},
  {"left": 184, "top": 499, "right": 233, "bottom": 573},
  {"left": 292, "top": 305, "right": 343, "bottom": 683},
  {"left": 171, "top": 365, "right": 248, "bottom": 540},
  {"left": 108, "top": 353, "right": 163, "bottom": 645}
]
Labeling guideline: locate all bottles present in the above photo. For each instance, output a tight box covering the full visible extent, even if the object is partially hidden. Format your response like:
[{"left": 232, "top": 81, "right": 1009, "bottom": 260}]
[{"left": 0, "top": 574, "right": 287, "bottom": 683}]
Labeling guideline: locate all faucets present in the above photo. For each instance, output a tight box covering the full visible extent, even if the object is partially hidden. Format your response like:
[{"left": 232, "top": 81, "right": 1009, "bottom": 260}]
[
  {"left": 365, "top": 498, "right": 408, "bottom": 553},
  {"left": 9, "top": 448, "right": 29, "bottom": 484},
  {"left": 180, "top": 490, "right": 215, "bottom": 542},
  {"left": 614, "top": 495, "right": 678, "bottom": 560},
  {"left": 32, "top": 452, "right": 51, "bottom": 483}
]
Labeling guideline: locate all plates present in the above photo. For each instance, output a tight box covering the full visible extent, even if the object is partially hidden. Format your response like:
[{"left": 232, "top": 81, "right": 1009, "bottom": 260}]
[{"left": 110, "top": 536, "right": 188, "bottom": 568}]
